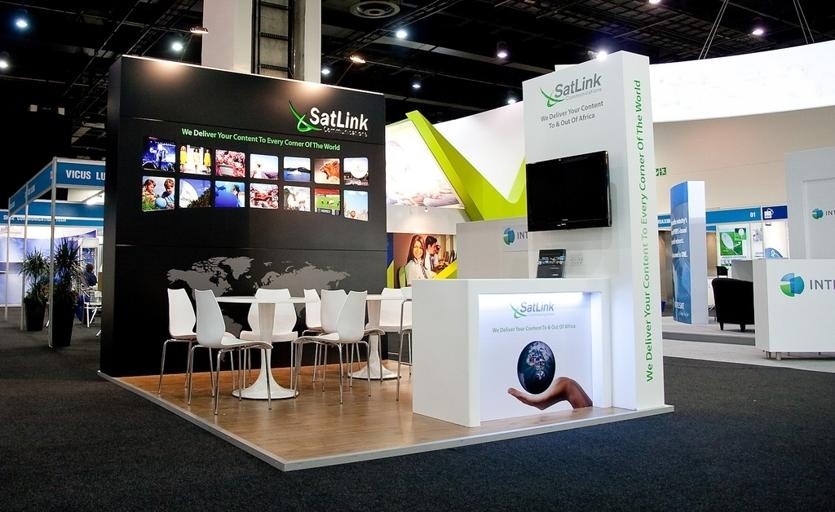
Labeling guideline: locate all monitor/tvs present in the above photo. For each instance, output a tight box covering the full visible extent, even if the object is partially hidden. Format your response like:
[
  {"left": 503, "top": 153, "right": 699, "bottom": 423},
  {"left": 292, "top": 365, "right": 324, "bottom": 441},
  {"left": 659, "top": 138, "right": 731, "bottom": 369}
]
[{"left": 525, "top": 150, "right": 614, "bottom": 233}]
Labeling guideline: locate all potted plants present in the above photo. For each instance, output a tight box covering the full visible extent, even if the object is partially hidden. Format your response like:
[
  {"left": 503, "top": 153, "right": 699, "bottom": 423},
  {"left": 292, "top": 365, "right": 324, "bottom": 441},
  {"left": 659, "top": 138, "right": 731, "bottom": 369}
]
[{"left": 16, "top": 237, "right": 93, "bottom": 348}]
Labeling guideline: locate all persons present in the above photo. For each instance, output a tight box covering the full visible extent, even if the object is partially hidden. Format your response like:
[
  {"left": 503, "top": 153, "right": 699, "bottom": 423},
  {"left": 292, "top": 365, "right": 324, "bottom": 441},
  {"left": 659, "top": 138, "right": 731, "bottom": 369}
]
[
  {"left": 143, "top": 178, "right": 158, "bottom": 206},
  {"left": 507, "top": 378, "right": 593, "bottom": 412},
  {"left": 193, "top": 147, "right": 200, "bottom": 172},
  {"left": 179, "top": 146, "right": 188, "bottom": 172},
  {"left": 83, "top": 263, "right": 98, "bottom": 287},
  {"left": 404, "top": 233, "right": 427, "bottom": 283},
  {"left": 161, "top": 178, "right": 173, "bottom": 211},
  {"left": 424, "top": 236, "right": 441, "bottom": 273},
  {"left": 204, "top": 149, "right": 212, "bottom": 174}
]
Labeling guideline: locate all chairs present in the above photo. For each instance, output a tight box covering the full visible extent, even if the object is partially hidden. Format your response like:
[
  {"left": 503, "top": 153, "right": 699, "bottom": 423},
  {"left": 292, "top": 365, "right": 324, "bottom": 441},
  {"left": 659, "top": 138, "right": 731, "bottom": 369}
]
[
  {"left": 712, "top": 277, "right": 755, "bottom": 332},
  {"left": 158, "top": 287, "right": 412, "bottom": 415}
]
